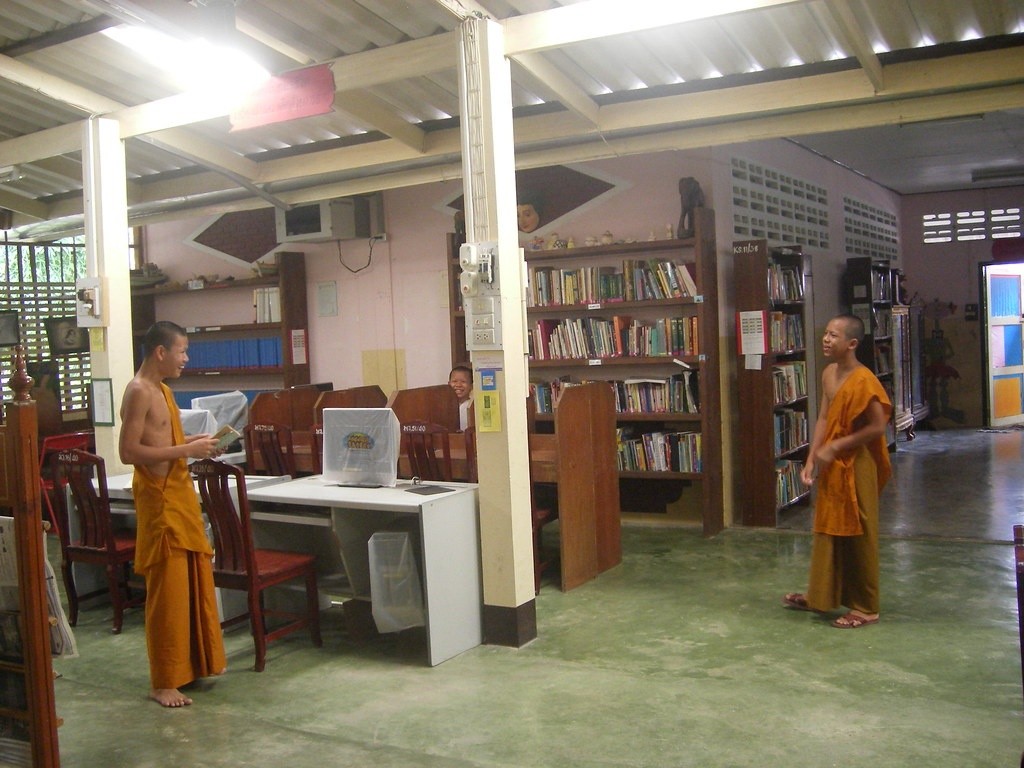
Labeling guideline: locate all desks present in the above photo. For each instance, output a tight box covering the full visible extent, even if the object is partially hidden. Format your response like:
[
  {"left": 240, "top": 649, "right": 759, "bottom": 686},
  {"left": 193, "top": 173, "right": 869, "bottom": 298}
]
[
  {"left": 63, "top": 470, "right": 292, "bottom": 636},
  {"left": 239, "top": 470, "right": 486, "bottom": 670}
]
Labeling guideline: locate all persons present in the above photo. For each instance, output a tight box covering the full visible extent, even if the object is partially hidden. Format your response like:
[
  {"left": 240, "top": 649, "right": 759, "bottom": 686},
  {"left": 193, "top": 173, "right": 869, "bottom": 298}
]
[
  {"left": 448, "top": 366, "right": 473, "bottom": 431},
  {"left": 518, "top": 196, "right": 542, "bottom": 233},
  {"left": 784, "top": 315, "right": 892, "bottom": 628},
  {"left": 119, "top": 320, "right": 228, "bottom": 707}
]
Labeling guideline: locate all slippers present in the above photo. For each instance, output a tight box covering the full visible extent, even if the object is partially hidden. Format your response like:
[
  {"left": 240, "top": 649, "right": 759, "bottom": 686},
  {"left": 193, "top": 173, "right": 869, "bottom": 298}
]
[
  {"left": 830, "top": 610, "right": 880, "bottom": 628},
  {"left": 783, "top": 592, "right": 826, "bottom": 612}
]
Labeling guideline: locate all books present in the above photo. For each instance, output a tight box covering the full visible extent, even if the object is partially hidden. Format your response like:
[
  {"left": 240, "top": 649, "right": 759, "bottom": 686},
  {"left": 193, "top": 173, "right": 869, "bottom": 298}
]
[
  {"left": 527, "top": 316, "right": 699, "bottom": 360},
  {"left": 770, "top": 311, "right": 805, "bottom": 351},
  {"left": 768, "top": 264, "right": 803, "bottom": 300},
  {"left": 770, "top": 361, "right": 807, "bottom": 403},
  {"left": 529, "top": 358, "right": 700, "bottom": 414},
  {"left": 617, "top": 426, "right": 702, "bottom": 473},
  {"left": 525, "top": 258, "right": 697, "bottom": 308},
  {"left": 874, "top": 270, "right": 894, "bottom": 402},
  {"left": 207, "top": 425, "right": 240, "bottom": 458},
  {"left": 774, "top": 408, "right": 807, "bottom": 456},
  {"left": 254, "top": 287, "right": 282, "bottom": 324},
  {"left": 775, "top": 460, "right": 809, "bottom": 506},
  {"left": 138, "top": 337, "right": 282, "bottom": 368}
]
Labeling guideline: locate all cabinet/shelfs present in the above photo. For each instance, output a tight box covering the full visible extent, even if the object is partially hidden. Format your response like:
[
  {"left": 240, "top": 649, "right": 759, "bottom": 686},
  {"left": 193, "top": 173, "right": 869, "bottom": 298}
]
[
  {"left": 129, "top": 250, "right": 310, "bottom": 416},
  {"left": 446, "top": 204, "right": 726, "bottom": 537},
  {"left": 853, "top": 298, "right": 939, "bottom": 454},
  {"left": 731, "top": 240, "right": 819, "bottom": 527}
]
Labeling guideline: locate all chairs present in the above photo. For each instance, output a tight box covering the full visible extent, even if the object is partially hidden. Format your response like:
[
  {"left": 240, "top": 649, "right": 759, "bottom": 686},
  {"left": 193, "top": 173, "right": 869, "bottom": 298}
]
[
  {"left": 185, "top": 455, "right": 324, "bottom": 674},
  {"left": 242, "top": 417, "right": 477, "bottom": 484},
  {"left": 48, "top": 447, "right": 145, "bottom": 636}
]
